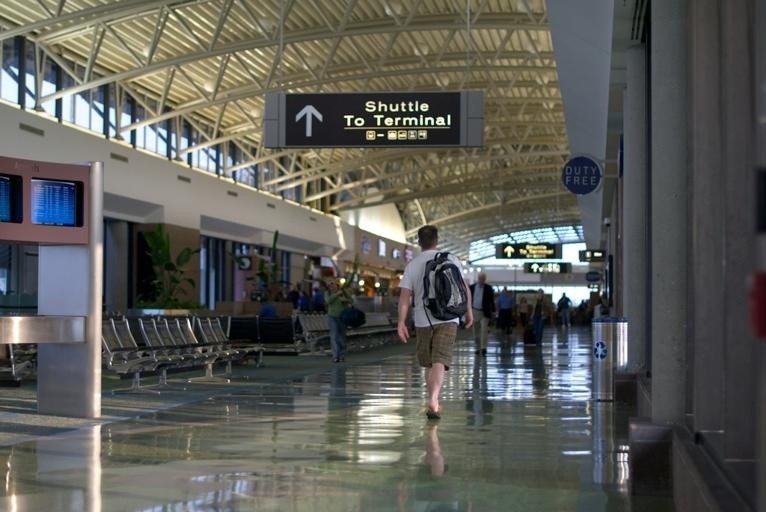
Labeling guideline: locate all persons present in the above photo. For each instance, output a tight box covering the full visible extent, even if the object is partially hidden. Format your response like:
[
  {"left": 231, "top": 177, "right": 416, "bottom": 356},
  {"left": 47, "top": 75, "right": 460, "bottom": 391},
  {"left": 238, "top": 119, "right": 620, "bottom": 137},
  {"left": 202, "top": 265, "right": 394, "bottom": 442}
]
[
  {"left": 323, "top": 277, "right": 354, "bottom": 363},
  {"left": 397, "top": 224, "right": 473, "bottom": 420},
  {"left": 468, "top": 273, "right": 497, "bottom": 357},
  {"left": 395, "top": 422, "right": 475, "bottom": 512},
  {"left": 275, "top": 282, "right": 327, "bottom": 314},
  {"left": 492, "top": 285, "right": 606, "bottom": 348}
]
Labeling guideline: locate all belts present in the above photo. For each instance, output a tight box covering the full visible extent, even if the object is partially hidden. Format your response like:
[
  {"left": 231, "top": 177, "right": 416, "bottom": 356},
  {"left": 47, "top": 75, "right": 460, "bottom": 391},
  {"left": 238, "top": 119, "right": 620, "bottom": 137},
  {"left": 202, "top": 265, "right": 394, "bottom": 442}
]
[{"left": 473, "top": 307, "right": 482, "bottom": 311}]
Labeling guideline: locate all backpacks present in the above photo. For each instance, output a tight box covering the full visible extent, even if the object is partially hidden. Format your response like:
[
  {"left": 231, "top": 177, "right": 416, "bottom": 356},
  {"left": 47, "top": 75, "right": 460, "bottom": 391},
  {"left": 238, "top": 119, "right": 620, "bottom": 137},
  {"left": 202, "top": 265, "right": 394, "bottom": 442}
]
[{"left": 422, "top": 252, "right": 466, "bottom": 320}]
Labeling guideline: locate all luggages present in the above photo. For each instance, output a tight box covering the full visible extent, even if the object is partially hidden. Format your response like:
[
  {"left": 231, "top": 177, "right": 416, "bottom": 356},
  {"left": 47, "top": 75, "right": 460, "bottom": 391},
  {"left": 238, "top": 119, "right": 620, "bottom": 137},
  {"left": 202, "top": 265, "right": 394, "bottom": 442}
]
[{"left": 524, "top": 324, "right": 535, "bottom": 344}]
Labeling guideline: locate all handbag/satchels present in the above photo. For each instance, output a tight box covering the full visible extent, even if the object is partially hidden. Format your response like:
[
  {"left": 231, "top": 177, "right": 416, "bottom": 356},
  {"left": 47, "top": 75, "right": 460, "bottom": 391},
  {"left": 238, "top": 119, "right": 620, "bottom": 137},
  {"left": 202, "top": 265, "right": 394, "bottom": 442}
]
[{"left": 341, "top": 308, "right": 366, "bottom": 328}]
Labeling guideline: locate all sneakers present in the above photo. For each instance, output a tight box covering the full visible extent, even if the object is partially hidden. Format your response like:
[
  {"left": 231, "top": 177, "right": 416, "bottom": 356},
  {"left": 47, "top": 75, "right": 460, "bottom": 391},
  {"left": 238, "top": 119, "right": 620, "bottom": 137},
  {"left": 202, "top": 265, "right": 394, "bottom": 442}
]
[{"left": 333, "top": 356, "right": 345, "bottom": 363}]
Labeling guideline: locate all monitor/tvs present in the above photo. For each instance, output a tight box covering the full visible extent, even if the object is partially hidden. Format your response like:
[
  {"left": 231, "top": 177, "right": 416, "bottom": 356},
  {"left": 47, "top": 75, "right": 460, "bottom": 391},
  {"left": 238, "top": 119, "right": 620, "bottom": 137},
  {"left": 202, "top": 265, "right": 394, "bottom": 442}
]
[
  {"left": 0, "top": 175, "right": 18, "bottom": 222},
  {"left": 30, "top": 178, "right": 82, "bottom": 227}
]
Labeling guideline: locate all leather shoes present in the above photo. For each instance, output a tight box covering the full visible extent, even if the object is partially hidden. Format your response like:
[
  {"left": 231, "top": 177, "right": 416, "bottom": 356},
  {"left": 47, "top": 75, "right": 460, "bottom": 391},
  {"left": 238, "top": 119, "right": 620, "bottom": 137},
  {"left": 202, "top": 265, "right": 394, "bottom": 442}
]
[{"left": 475, "top": 348, "right": 486, "bottom": 355}]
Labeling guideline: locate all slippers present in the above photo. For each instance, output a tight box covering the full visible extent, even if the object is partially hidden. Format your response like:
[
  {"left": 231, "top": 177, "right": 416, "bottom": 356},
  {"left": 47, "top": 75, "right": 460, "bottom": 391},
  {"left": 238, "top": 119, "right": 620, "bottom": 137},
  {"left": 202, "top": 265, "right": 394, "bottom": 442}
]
[{"left": 426, "top": 410, "right": 441, "bottom": 419}]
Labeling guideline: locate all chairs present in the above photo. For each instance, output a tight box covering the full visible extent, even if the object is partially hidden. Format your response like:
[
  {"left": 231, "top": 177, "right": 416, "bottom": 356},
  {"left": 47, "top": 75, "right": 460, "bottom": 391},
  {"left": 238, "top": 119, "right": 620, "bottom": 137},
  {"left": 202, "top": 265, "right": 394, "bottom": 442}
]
[
  {"left": 0, "top": 344, "right": 33, "bottom": 387},
  {"left": 101, "top": 312, "right": 398, "bottom": 396}
]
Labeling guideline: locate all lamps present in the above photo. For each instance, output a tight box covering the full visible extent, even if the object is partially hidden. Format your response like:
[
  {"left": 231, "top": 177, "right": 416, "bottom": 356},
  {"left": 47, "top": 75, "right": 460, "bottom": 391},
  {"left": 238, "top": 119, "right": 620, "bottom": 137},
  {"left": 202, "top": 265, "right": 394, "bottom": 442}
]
[{"left": 339, "top": 260, "right": 403, "bottom": 288}]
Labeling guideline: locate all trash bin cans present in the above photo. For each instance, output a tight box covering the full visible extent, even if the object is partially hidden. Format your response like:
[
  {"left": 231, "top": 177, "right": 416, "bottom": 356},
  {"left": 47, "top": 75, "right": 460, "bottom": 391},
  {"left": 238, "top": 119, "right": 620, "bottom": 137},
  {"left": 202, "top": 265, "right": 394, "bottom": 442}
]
[{"left": 591, "top": 316, "right": 630, "bottom": 402}]
